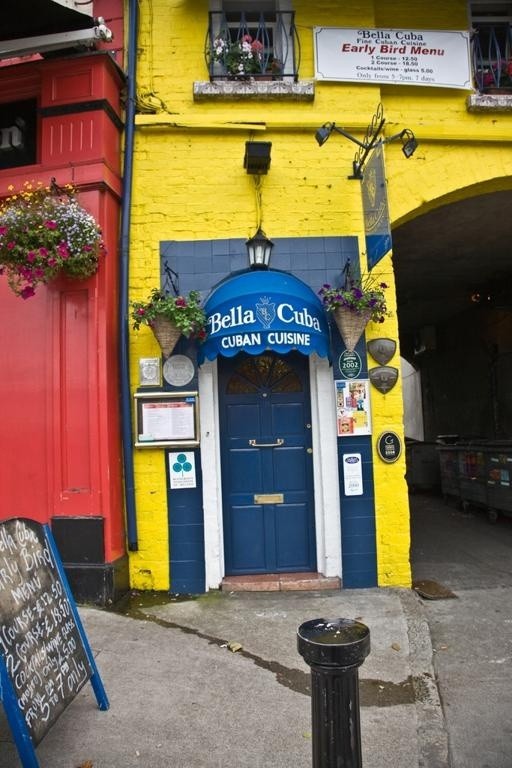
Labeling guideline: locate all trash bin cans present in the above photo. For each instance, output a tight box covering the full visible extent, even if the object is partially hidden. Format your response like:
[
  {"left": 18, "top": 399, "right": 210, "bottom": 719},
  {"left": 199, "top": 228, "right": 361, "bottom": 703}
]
[{"left": 404, "top": 435, "right": 512, "bottom": 521}]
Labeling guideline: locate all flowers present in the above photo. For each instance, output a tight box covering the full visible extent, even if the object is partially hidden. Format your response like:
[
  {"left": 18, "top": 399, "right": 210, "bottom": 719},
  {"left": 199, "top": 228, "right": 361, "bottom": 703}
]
[
  {"left": 207, "top": 32, "right": 264, "bottom": 80},
  {"left": 1, "top": 176, "right": 108, "bottom": 301},
  {"left": 473, "top": 54, "right": 511, "bottom": 93},
  {"left": 125, "top": 288, "right": 209, "bottom": 344},
  {"left": 313, "top": 264, "right": 391, "bottom": 323}
]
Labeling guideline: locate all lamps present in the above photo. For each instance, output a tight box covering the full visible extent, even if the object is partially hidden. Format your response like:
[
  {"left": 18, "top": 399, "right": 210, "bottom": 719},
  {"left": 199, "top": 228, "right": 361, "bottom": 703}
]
[
  {"left": 316, "top": 103, "right": 422, "bottom": 183},
  {"left": 242, "top": 225, "right": 275, "bottom": 268}
]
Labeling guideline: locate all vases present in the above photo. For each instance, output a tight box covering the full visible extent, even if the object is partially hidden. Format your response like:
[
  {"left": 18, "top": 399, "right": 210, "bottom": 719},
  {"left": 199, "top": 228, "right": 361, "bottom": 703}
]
[
  {"left": 148, "top": 317, "right": 183, "bottom": 359},
  {"left": 330, "top": 304, "right": 370, "bottom": 355}
]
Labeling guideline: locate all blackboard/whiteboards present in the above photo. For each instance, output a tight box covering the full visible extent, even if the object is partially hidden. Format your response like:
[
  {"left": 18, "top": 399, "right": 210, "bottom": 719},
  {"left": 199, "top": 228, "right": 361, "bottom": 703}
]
[{"left": 0, "top": 517, "right": 95, "bottom": 749}]
